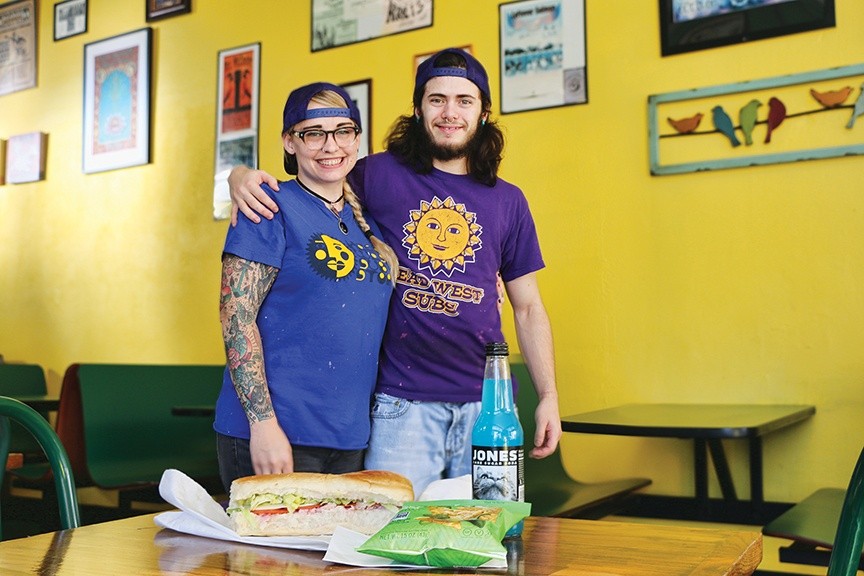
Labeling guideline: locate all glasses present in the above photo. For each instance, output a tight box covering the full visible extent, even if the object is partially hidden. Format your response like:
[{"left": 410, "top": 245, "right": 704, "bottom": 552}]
[{"left": 290, "top": 125, "right": 362, "bottom": 151}]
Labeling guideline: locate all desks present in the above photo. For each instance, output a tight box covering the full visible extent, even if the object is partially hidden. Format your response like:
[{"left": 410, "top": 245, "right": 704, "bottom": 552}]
[
  {"left": 560, "top": 404, "right": 813, "bottom": 522},
  {"left": 0, "top": 506, "right": 763, "bottom": 576}
]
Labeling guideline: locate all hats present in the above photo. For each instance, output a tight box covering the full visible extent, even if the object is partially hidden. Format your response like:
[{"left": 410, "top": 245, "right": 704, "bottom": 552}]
[
  {"left": 283, "top": 82, "right": 362, "bottom": 175},
  {"left": 414, "top": 49, "right": 491, "bottom": 101}
]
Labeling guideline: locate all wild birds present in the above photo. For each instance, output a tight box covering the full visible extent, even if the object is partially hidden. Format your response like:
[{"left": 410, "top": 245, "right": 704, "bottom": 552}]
[
  {"left": 711, "top": 106, "right": 741, "bottom": 147},
  {"left": 765, "top": 97, "right": 785, "bottom": 143},
  {"left": 809, "top": 87, "right": 854, "bottom": 108},
  {"left": 846, "top": 82, "right": 864, "bottom": 129},
  {"left": 667, "top": 113, "right": 704, "bottom": 132},
  {"left": 741, "top": 100, "right": 763, "bottom": 145}
]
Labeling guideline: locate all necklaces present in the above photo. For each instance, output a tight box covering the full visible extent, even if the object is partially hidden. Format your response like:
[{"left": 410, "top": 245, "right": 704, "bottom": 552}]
[
  {"left": 338, "top": 219, "right": 349, "bottom": 235},
  {"left": 296, "top": 174, "right": 345, "bottom": 219}
]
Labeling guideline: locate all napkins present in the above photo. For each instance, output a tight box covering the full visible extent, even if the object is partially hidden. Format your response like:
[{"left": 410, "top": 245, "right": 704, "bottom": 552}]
[{"left": 156, "top": 469, "right": 505, "bottom": 569}]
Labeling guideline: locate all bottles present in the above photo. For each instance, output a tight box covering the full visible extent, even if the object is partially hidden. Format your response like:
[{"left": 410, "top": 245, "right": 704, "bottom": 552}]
[{"left": 471, "top": 341, "right": 524, "bottom": 539}]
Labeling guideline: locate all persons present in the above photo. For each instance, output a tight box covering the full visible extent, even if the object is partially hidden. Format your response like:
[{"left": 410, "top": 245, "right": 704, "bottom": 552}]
[
  {"left": 213, "top": 83, "right": 400, "bottom": 490},
  {"left": 226, "top": 48, "right": 563, "bottom": 501}
]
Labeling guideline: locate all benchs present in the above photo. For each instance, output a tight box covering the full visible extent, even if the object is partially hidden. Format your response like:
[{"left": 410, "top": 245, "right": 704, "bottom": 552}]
[
  {"left": 0, "top": 364, "right": 227, "bottom": 506},
  {"left": 510, "top": 361, "right": 652, "bottom": 518},
  {"left": 764, "top": 487, "right": 864, "bottom": 573}
]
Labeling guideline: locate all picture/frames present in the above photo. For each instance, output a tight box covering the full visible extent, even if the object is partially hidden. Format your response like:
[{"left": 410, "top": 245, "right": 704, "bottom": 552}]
[
  {"left": 659, "top": 0, "right": 835, "bottom": 56},
  {"left": 342, "top": 78, "right": 373, "bottom": 159},
  {"left": 213, "top": 42, "right": 260, "bottom": 220},
  {"left": 146, "top": 0, "right": 190, "bottom": 22},
  {"left": 0, "top": 0, "right": 37, "bottom": 96},
  {"left": 53, "top": 1, "right": 88, "bottom": 41},
  {"left": 499, "top": 1, "right": 588, "bottom": 115},
  {"left": 82, "top": 26, "right": 152, "bottom": 175},
  {"left": 311, "top": 1, "right": 433, "bottom": 53}
]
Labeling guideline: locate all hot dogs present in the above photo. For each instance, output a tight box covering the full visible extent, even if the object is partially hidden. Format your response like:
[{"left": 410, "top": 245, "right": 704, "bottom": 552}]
[{"left": 227, "top": 469, "right": 415, "bottom": 536}]
